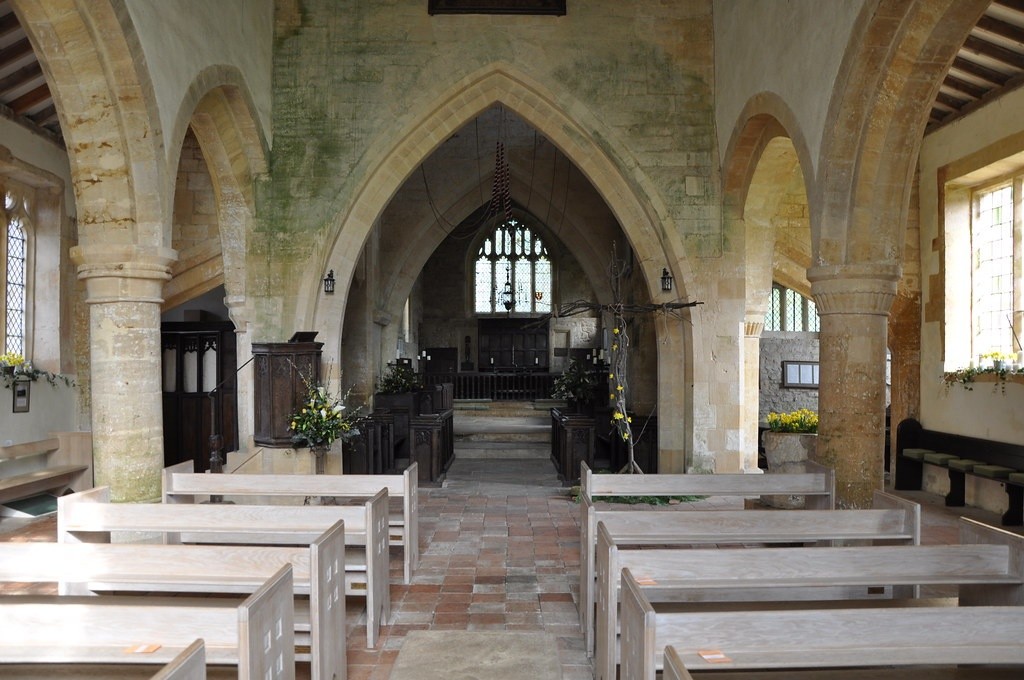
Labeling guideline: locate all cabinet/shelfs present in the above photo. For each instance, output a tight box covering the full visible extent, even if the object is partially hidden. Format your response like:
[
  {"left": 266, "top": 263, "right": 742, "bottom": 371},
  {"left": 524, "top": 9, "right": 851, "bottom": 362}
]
[{"left": 160, "top": 321, "right": 238, "bottom": 473}]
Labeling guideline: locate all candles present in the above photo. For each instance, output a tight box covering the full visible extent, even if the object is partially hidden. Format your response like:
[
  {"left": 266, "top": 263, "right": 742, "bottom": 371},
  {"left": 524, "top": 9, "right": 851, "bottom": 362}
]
[
  {"left": 490, "top": 358, "right": 492, "bottom": 364},
  {"left": 535, "top": 358, "right": 537, "bottom": 364},
  {"left": 586, "top": 349, "right": 610, "bottom": 364},
  {"left": 387, "top": 348, "right": 430, "bottom": 363}
]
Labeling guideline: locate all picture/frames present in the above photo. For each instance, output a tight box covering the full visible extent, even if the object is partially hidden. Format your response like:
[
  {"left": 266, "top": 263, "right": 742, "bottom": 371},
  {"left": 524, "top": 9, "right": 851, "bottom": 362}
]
[{"left": 12, "top": 381, "right": 30, "bottom": 413}]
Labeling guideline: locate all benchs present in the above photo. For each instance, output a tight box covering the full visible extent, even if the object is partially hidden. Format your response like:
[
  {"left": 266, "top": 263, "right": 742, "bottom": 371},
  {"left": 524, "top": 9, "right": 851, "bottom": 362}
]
[
  {"left": 0, "top": 432, "right": 419, "bottom": 680},
  {"left": 577, "top": 416, "right": 1024, "bottom": 680}
]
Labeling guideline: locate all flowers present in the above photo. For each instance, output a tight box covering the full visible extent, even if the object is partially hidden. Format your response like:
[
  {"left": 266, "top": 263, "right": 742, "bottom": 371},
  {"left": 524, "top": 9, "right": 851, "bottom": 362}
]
[
  {"left": 0, "top": 351, "right": 24, "bottom": 367},
  {"left": 376, "top": 365, "right": 424, "bottom": 393},
  {"left": 982, "top": 352, "right": 1018, "bottom": 361},
  {"left": 551, "top": 362, "right": 601, "bottom": 404},
  {"left": 766, "top": 408, "right": 819, "bottom": 433},
  {"left": 286, "top": 357, "right": 369, "bottom": 458}
]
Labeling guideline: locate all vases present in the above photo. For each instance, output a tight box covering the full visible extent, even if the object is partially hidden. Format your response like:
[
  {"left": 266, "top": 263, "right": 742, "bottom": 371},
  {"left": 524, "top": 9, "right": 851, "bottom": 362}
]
[
  {"left": 1, "top": 366, "right": 15, "bottom": 375},
  {"left": 764, "top": 429, "right": 817, "bottom": 474},
  {"left": 994, "top": 359, "right": 1006, "bottom": 371},
  {"left": 567, "top": 399, "right": 585, "bottom": 412}
]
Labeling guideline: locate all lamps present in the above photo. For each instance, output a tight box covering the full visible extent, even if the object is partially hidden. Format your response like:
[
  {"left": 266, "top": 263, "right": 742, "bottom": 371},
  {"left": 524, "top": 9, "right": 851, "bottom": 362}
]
[
  {"left": 660, "top": 267, "right": 673, "bottom": 291},
  {"left": 503, "top": 268, "right": 513, "bottom": 311},
  {"left": 323, "top": 268, "right": 335, "bottom": 292}
]
